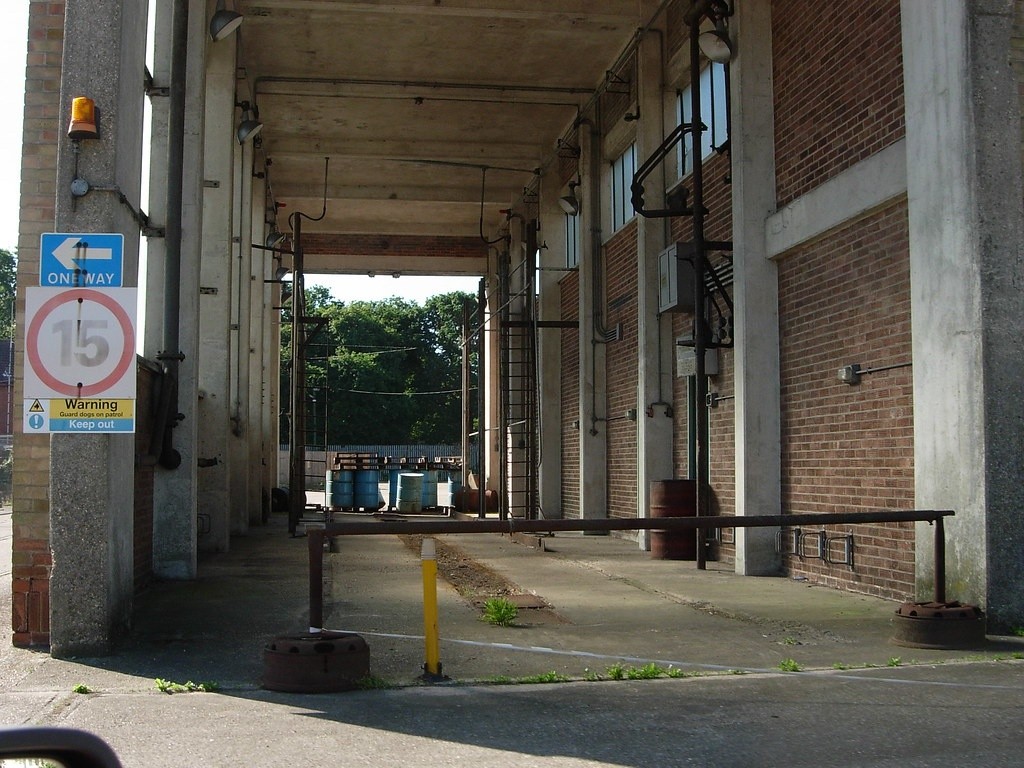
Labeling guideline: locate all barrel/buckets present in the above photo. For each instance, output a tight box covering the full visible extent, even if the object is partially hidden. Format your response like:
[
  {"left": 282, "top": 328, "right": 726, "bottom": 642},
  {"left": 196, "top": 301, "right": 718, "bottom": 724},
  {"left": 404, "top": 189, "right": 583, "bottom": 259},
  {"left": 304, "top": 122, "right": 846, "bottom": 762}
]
[
  {"left": 389, "top": 470, "right": 414, "bottom": 510},
  {"left": 395, "top": 473, "right": 424, "bottom": 514},
  {"left": 417, "top": 470, "right": 438, "bottom": 507},
  {"left": 649, "top": 480, "right": 696, "bottom": 560},
  {"left": 448, "top": 472, "right": 470, "bottom": 510},
  {"left": 468, "top": 489, "right": 499, "bottom": 513},
  {"left": 326, "top": 468, "right": 386, "bottom": 510}
]
